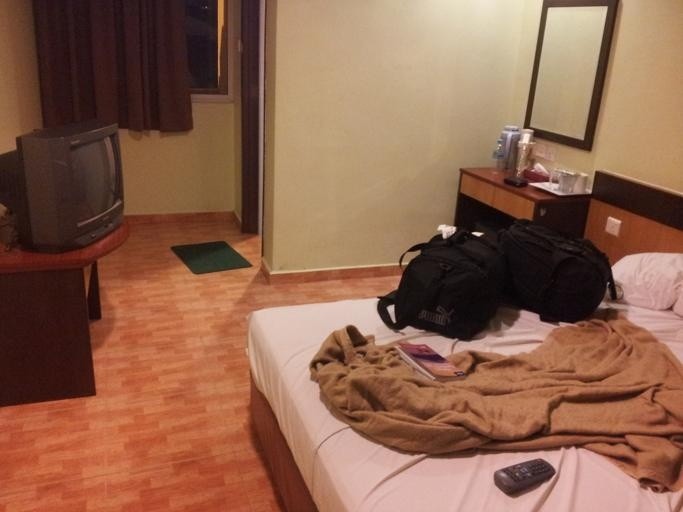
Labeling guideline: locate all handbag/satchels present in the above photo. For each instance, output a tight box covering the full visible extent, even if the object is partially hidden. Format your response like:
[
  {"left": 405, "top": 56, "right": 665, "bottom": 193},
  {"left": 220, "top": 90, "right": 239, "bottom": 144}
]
[{"left": 395, "top": 219, "right": 617, "bottom": 339}]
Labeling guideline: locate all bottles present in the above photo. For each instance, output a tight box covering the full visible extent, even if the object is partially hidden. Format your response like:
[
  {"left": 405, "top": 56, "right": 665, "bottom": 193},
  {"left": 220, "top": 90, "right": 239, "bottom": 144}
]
[{"left": 490, "top": 125, "right": 534, "bottom": 175}]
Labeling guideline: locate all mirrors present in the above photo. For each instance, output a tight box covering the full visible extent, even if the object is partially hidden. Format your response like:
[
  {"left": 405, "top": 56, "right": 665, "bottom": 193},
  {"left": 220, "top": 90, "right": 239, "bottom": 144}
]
[{"left": 523, "top": 0, "right": 619, "bottom": 151}]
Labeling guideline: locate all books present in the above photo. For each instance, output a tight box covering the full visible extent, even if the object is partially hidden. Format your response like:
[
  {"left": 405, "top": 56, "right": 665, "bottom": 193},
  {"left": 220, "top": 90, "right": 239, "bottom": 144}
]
[{"left": 393, "top": 340, "right": 466, "bottom": 384}]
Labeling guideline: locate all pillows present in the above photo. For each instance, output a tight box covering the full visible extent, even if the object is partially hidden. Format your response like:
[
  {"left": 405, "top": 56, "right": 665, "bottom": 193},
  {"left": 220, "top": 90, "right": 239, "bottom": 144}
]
[
  {"left": 244, "top": 170, "right": 683, "bottom": 512},
  {"left": 672, "top": 297, "right": 683, "bottom": 319},
  {"left": 603, "top": 252, "right": 683, "bottom": 311}
]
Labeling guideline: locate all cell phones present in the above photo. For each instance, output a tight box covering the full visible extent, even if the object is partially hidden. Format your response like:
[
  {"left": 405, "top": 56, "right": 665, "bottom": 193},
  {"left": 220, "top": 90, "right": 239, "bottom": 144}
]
[{"left": 503, "top": 177, "right": 528, "bottom": 187}]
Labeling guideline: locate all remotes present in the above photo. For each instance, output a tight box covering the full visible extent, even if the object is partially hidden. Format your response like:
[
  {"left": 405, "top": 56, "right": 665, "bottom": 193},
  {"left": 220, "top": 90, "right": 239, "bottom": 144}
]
[{"left": 493, "top": 459, "right": 556, "bottom": 495}]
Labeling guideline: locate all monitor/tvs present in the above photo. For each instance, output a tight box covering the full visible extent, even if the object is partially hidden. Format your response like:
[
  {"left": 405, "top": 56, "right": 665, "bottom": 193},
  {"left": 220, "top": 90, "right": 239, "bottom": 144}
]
[{"left": 0, "top": 119, "right": 124, "bottom": 254}]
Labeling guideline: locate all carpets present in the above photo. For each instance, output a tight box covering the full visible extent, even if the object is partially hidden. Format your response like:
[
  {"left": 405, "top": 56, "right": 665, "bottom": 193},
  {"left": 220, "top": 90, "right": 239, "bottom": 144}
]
[{"left": 171, "top": 240, "right": 253, "bottom": 274}]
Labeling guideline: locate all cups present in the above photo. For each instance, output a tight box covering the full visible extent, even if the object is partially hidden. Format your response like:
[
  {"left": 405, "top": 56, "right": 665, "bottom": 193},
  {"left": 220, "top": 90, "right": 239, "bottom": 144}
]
[{"left": 549, "top": 168, "right": 590, "bottom": 194}]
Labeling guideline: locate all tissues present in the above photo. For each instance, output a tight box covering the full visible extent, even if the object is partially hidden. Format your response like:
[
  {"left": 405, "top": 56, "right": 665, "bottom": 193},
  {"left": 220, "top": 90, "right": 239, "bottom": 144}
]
[{"left": 524, "top": 163, "right": 550, "bottom": 181}]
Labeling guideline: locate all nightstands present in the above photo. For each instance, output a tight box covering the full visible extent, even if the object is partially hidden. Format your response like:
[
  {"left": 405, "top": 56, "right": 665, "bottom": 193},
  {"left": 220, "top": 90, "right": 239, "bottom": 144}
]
[{"left": 453, "top": 167, "right": 591, "bottom": 239}]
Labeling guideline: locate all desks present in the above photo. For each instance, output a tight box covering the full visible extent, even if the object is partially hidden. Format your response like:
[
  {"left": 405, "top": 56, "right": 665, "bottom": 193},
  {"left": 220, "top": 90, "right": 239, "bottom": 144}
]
[{"left": 0, "top": 216, "right": 129, "bottom": 408}]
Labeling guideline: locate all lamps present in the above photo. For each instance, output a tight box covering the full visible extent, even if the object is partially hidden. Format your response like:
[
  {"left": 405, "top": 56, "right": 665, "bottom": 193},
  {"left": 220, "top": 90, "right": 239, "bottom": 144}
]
[{"left": 514, "top": 129, "right": 536, "bottom": 178}]
